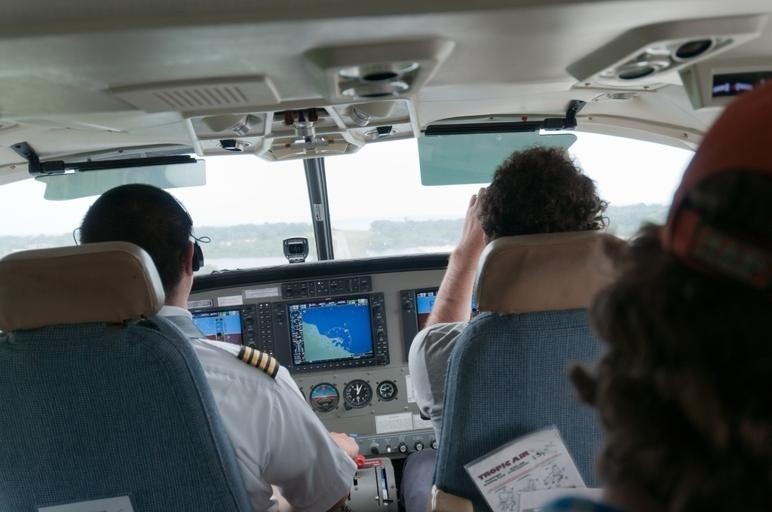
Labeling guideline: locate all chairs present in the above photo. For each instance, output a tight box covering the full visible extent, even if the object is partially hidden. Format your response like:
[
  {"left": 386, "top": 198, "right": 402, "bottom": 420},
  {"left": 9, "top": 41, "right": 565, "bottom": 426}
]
[
  {"left": 428, "top": 231, "right": 630, "bottom": 512},
  {"left": 0, "top": 240, "right": 255, "bottom": 512}
]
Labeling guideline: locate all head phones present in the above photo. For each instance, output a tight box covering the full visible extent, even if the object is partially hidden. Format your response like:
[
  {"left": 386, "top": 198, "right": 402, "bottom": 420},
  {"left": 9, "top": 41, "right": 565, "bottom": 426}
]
[{"left": 80, "top": 183, "right": 204, "bottom": 271}]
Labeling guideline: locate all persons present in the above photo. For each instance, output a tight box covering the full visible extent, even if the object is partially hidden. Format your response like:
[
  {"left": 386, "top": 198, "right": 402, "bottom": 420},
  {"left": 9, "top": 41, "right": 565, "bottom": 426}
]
[
  {"left": 397, "top": 144, "right": 612, "bottom": 511},
  {"left": 532, "top": 72, "right": 772, "bottom": 511},
  {"left": 80, "top": 183, "right": 364, "bottom": 511}
]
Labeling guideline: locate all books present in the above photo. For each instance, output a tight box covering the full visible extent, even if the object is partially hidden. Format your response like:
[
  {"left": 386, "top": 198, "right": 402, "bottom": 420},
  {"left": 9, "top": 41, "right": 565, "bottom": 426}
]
[{"left": 464, "top": 424, "right": 588, "bottom": 511}]
